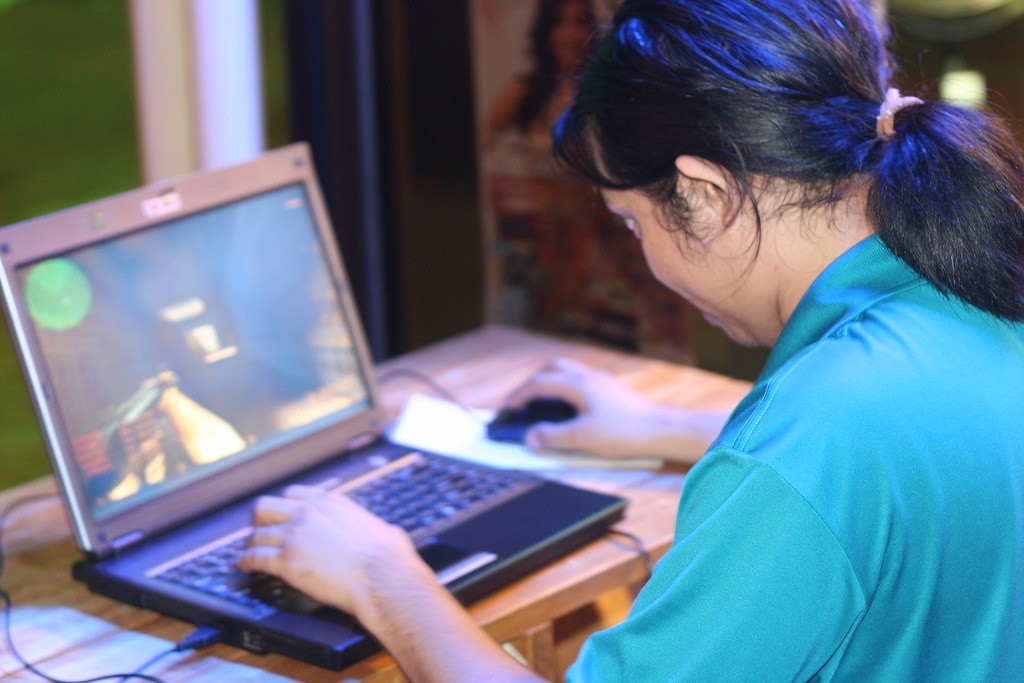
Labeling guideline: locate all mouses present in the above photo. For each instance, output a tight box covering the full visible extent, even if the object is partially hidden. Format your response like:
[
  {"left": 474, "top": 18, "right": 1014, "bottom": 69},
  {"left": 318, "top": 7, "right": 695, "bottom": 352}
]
[{"left": 488, "top": 397, "right": 576, "bottom": 445}]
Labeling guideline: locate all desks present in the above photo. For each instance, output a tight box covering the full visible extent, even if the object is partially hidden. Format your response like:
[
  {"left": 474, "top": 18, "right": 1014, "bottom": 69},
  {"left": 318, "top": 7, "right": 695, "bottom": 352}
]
[{"left": 0, "top": 322, "right": 756, "bottom": 683}]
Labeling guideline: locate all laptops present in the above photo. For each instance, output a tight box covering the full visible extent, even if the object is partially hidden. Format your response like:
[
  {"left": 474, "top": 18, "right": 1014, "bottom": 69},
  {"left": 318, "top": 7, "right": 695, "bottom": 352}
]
[{"left": 0, "top": 142, "right": 624, "bottom": 671}]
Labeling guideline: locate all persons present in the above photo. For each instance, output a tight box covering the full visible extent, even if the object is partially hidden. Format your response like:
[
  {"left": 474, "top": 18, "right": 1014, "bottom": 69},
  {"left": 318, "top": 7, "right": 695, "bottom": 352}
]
[{"left": 240, "top": 0, "right": 1024, "bottom": 683}]
